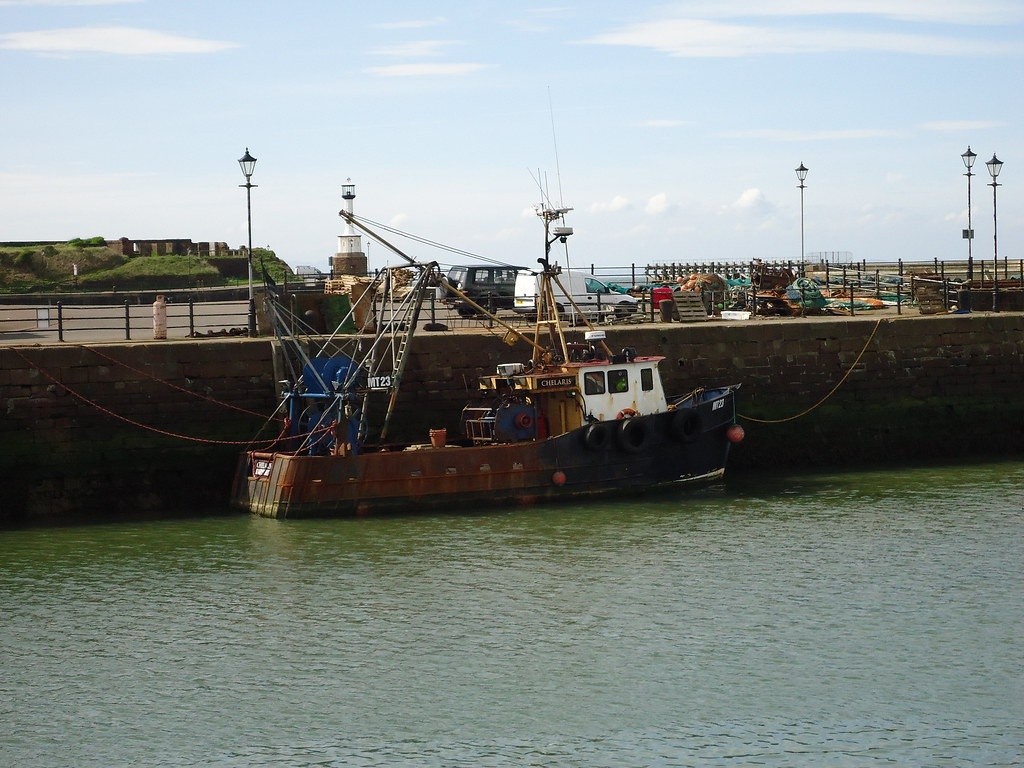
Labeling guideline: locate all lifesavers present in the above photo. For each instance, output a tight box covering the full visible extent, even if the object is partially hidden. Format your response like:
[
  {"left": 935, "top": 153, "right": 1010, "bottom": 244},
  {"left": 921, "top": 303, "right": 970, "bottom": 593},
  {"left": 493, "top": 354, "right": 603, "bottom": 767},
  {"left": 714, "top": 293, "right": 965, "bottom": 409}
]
[{"left": 616, "top": 408, "right": 638, "bottom": 420}]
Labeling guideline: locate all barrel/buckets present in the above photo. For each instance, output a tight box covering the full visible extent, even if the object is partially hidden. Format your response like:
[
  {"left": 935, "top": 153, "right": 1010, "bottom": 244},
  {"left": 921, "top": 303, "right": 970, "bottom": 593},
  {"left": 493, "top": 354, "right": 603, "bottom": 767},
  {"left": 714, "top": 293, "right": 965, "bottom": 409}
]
[{"left": 429, "top": 428, "right": 446, "bottom": 447}]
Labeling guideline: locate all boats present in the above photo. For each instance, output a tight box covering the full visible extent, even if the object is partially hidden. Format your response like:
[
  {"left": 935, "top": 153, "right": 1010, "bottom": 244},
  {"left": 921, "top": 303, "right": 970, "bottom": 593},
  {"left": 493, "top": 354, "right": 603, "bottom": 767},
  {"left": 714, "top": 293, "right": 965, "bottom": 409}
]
[{"left": 233, "top": 166, "right": 752, "bottom": 518}]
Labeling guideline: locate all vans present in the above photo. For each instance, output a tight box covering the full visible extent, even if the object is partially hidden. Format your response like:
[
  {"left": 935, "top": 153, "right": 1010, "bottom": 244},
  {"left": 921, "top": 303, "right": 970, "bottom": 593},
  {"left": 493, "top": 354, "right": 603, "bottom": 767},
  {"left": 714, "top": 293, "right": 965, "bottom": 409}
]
[{"left": 514, "top": 268, "right": 638, "bottom": 322}]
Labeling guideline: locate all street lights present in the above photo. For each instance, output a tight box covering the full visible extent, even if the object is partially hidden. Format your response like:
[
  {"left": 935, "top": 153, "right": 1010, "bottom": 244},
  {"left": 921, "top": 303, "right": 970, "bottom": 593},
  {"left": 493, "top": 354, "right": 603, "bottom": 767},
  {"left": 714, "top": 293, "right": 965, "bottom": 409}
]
[
  {"left": 961, "top": 146, "right": 977, "bottom": 279},
  {"left": 796, "top": 162, "right": 808, "bottom": 270},
  {"left": 367, "top": 241, "right": 371, "bottom": 270},
  {"left": 40, "top": 248, "right": 45, "bottom": 290},
  {"left": 238, "top": 147, "right": 259, "bottom": 338},
  {"left": 985, "top": 153, "right": 1004, "bottom": 312}
]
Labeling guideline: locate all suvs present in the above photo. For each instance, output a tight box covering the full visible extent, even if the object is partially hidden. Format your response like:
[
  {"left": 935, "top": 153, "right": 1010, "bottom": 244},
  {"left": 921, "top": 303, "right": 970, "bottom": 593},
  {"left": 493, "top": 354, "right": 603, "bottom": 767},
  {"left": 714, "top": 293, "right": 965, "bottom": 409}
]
[{"left": 442, "top": 263, "right": 531, "bottom": 317}]
